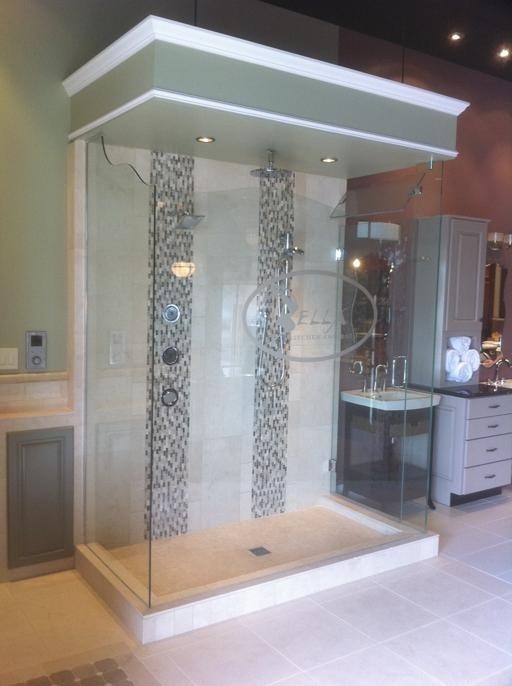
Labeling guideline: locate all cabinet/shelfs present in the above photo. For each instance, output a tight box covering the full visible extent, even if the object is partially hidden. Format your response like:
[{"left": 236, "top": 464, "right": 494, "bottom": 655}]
[{"left": 407, "top": 215, "right": 512, "bottom": 507}]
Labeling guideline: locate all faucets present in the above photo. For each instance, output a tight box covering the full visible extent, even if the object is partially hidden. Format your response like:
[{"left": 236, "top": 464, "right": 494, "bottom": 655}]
[
  {"left": 374, "top": 364, "right": 388, "bottom": 389},
  {"left": 494, "top": 359, "right": 512, "bottom": 381}
]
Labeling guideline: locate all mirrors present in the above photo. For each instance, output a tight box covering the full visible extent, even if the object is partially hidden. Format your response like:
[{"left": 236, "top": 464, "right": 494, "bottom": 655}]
[{"left": 351, "top": 254, "right": 391, "bottom": 377}]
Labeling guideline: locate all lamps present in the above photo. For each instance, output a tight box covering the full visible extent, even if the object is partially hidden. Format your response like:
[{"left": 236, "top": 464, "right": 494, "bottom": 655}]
[{"left": 356, "top": 221, "right": 401, "bottom": 252}]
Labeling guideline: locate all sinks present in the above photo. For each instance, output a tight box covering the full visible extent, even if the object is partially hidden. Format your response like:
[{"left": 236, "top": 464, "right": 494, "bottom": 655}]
[{"left": 366, "top": 391, "right": 424, "bottom": 400}]
